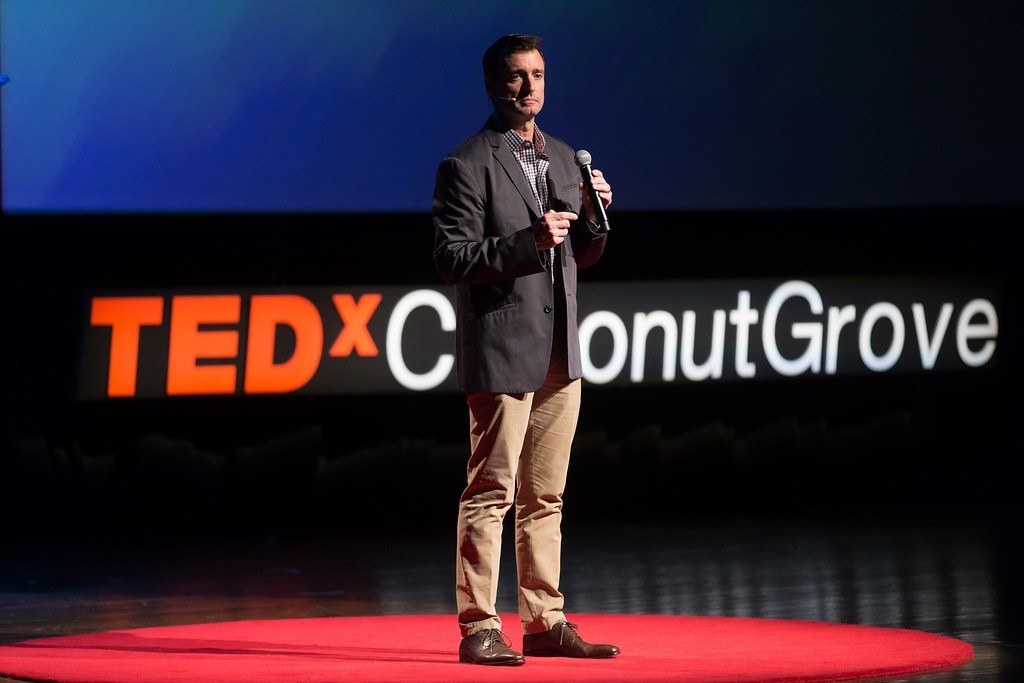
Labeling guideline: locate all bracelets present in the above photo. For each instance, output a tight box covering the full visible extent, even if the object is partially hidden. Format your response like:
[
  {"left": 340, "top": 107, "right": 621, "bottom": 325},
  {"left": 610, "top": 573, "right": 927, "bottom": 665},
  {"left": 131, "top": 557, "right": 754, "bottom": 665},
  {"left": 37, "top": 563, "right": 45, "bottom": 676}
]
[{"left": 586, "top": 218, "right": 601, "bottom": 232}]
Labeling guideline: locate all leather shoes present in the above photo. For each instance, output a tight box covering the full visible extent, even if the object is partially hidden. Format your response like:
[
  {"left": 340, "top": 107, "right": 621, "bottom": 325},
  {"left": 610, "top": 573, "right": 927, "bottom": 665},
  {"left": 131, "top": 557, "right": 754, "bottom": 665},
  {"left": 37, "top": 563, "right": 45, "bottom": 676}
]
[
  {"left": 459, "top": 628, "right": 526, "bottom": 664},
  {"left": 522, "top": 621, "right": 620, "bottom": 658}
]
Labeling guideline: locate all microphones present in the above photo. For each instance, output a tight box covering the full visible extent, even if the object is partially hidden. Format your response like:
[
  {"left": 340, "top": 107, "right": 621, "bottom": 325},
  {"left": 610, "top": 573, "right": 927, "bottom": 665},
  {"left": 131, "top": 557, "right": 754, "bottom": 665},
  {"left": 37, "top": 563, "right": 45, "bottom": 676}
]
[
  {"left": 491, "top": 96, "right": 516, "bottom": 102},
  {"left": 574, "top": 150, "right": 612, "bottom": 233}
]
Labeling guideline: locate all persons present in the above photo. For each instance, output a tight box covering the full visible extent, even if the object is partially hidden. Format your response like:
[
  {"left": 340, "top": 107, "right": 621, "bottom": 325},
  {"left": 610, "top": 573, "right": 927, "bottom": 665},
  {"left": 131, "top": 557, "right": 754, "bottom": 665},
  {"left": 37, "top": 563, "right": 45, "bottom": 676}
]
[{"left": 430, "top": 32, "right": 621, "bottom": 664}]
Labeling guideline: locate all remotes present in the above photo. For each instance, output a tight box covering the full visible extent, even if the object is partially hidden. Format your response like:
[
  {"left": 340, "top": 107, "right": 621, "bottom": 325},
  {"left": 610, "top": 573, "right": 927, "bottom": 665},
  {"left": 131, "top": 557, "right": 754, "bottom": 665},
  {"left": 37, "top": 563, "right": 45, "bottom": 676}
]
[{"left": 556, "top": 201, "right": 573, "bottom": 212}]
